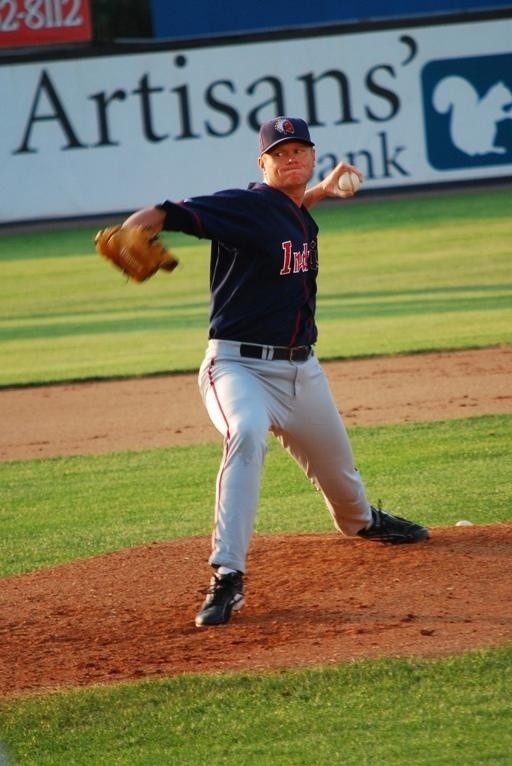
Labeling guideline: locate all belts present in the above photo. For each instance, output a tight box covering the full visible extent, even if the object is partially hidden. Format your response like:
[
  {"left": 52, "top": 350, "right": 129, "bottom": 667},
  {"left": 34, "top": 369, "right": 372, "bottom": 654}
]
[{"left": 240, "top": 344, "right": 312, "bottom": 361}]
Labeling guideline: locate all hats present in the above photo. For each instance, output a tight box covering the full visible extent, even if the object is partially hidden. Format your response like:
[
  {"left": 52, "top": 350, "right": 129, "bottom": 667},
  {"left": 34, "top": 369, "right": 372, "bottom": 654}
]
[{"left": 258, "top": 116, "right": 315, "bottom": 155}]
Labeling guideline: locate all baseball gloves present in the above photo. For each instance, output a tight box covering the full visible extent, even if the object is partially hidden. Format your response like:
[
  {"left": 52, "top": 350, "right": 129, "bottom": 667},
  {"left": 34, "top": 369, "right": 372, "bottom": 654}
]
[{"left": 94, "top": 224, "right": 177, "bottom": 283}]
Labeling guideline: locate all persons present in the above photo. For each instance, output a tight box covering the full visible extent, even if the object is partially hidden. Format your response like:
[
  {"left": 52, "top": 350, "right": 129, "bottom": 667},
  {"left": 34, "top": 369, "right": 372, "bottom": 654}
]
[{"left": 121, "top": 116, "right": 428, "bottom": 628}]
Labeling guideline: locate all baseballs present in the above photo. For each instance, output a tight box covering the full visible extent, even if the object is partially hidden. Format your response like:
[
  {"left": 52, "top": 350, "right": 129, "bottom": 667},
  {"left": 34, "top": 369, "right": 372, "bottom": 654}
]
[{"left": 339, "top": 171, "right": 360, "bottom": 192}]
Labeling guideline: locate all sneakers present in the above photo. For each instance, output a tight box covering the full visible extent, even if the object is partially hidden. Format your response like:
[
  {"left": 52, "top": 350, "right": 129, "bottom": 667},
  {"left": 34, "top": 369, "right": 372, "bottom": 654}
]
[
  {"left": 195, "top": 572, "right": 244, "bottom": 627},
  {"left": 357, "top": 498, "right": 427, "bottom": 544}
]
